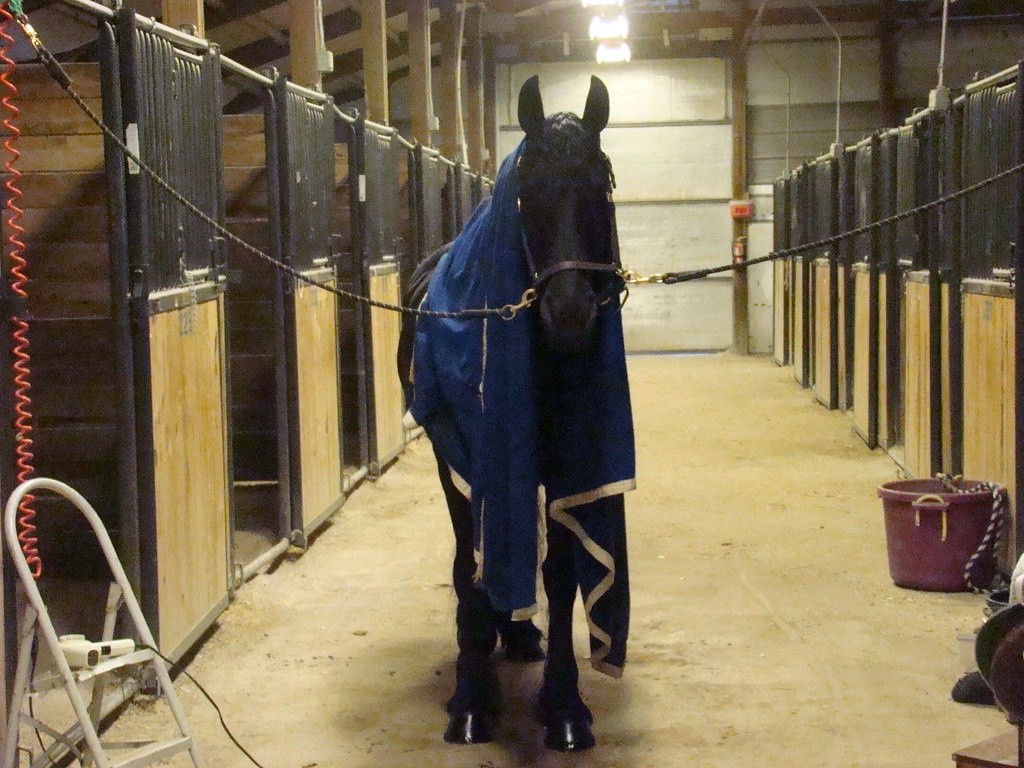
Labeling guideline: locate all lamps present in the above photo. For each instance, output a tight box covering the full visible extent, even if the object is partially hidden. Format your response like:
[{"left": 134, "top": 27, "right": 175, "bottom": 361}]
[
  {"left": 596, "top": 41, "right": 631, "bottom": 64},
  {"left": 581, "top": 0, "right": 625, "bottom": 11},
  {"left": 589, "top": 11, "right": 629, "bottom": 40}
]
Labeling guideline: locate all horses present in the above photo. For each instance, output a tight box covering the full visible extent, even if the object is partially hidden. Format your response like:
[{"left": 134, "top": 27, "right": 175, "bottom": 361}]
[{"left": 396, "top": 74, "right": 618, "bottom": 755}]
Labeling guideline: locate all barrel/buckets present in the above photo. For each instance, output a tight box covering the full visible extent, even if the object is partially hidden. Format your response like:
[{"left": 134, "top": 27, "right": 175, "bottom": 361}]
[{"left": 878, "top": 476, "right": 1005, "bottom": 591}]
